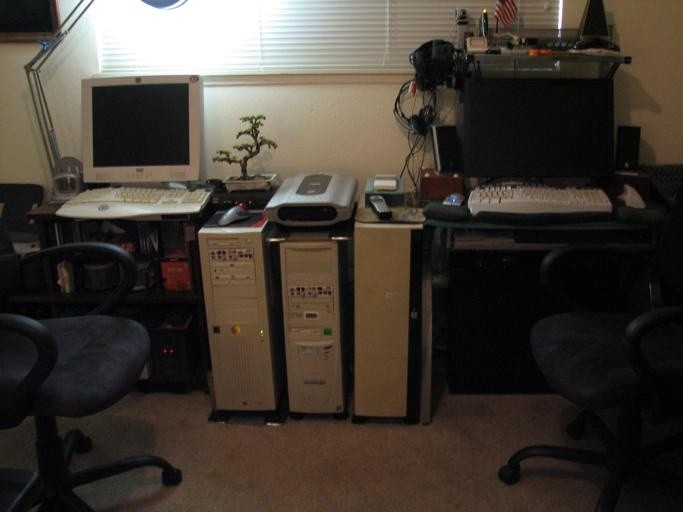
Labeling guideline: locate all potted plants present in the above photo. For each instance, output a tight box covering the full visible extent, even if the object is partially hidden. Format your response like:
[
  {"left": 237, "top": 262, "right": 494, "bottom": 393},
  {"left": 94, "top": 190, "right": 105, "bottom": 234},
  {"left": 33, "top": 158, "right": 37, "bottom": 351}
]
[{"left": 211, "top": 112, "right": 278, "bottom": 195}]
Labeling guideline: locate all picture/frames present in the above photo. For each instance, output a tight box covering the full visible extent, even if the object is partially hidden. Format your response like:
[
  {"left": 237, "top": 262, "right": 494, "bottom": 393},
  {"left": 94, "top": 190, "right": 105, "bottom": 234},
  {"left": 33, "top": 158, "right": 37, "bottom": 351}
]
[{"left": 0, "top": 0, "right": 62, "bottom": 42}]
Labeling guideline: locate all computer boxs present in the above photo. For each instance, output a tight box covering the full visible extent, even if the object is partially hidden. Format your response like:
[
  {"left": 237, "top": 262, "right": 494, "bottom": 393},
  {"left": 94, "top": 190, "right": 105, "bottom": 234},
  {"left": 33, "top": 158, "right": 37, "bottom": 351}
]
[
  {"left": 279, "top": 230, "right": 345, "bottom": 419},
  {"left": 197, "top": 210, "right": 281, "bottom": 415}
]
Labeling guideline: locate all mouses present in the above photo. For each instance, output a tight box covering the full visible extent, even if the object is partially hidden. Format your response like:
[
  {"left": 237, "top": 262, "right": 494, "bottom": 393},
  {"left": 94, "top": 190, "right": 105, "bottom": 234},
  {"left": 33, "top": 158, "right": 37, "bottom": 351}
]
[
  {"left": 440, "top": 191, "right": 463, "bottom": 206},
  {"left": 217, "top": 205, "right": 250, "bottom": 227}
]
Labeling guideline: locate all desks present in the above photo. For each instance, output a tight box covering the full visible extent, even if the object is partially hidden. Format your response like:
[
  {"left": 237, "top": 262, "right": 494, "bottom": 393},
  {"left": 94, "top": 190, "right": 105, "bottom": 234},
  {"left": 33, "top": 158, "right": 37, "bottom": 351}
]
[
  {"left": 24, "top": 182, "right": 279, "bottom": 395},
  {"left": 423, "top": 167, "right": 683, "bottom": 423}
]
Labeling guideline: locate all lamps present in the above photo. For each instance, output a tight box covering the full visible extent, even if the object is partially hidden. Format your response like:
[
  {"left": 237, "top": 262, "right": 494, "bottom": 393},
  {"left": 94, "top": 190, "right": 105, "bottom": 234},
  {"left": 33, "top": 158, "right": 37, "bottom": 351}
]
[{"left": 23, "top": 1, "right": 193, "bottom": 198}]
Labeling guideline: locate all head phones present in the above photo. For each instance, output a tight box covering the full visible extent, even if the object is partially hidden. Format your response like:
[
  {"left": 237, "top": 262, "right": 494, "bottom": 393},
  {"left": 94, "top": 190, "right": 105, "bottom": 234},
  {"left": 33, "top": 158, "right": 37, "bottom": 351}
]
[{"left": 393, "top": 79, "right": 435, "bottom": 135}]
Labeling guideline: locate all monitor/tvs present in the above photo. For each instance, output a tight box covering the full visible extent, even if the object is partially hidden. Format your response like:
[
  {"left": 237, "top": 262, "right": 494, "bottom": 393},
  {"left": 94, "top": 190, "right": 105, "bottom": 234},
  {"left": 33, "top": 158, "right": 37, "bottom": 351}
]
[
  {"left": 462, "top": 75, "right": 614, "bottom": 179},
  {"left": 81, "top": 76, "right": 200, "bottom": 187}
]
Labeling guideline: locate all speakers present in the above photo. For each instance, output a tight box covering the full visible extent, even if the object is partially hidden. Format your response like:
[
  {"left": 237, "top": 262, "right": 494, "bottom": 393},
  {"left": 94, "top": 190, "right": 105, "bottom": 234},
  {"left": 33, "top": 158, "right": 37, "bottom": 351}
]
[{"left": 615, "top": 125, "right": 641, "bottom": 172}]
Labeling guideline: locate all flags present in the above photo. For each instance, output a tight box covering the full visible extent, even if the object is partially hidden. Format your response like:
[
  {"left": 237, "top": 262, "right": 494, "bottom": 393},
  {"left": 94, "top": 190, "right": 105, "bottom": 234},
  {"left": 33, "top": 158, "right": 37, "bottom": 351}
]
[{"left": 495, "top": 0, "right": 519, "bottom": 29}]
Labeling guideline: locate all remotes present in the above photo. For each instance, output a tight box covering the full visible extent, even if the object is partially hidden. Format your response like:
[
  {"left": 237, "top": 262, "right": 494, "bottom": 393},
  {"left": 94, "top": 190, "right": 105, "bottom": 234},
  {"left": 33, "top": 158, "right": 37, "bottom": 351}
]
[{"left": 369, "top": 194, "right": 392, "bottom": 219}]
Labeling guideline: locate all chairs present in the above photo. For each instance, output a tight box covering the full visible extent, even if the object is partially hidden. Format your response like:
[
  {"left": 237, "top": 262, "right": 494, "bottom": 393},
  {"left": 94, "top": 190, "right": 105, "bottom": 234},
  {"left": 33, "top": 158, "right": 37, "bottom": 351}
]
[
  {"left": 498, "top": 180, "right": 683, "bottom": 511},
  {"left": 1, "top": 241, "right": 181, "bottom": 512}
]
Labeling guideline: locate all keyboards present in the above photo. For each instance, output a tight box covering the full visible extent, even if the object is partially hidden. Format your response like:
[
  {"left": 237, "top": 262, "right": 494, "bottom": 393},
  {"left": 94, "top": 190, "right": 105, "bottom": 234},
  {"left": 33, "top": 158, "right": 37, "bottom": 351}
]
[
  {"left": 53, "top": 187, "right": 213, "bottom": 221},
  {"left": 468, "top": 182, "right": 614, "bottom": 220}
]
